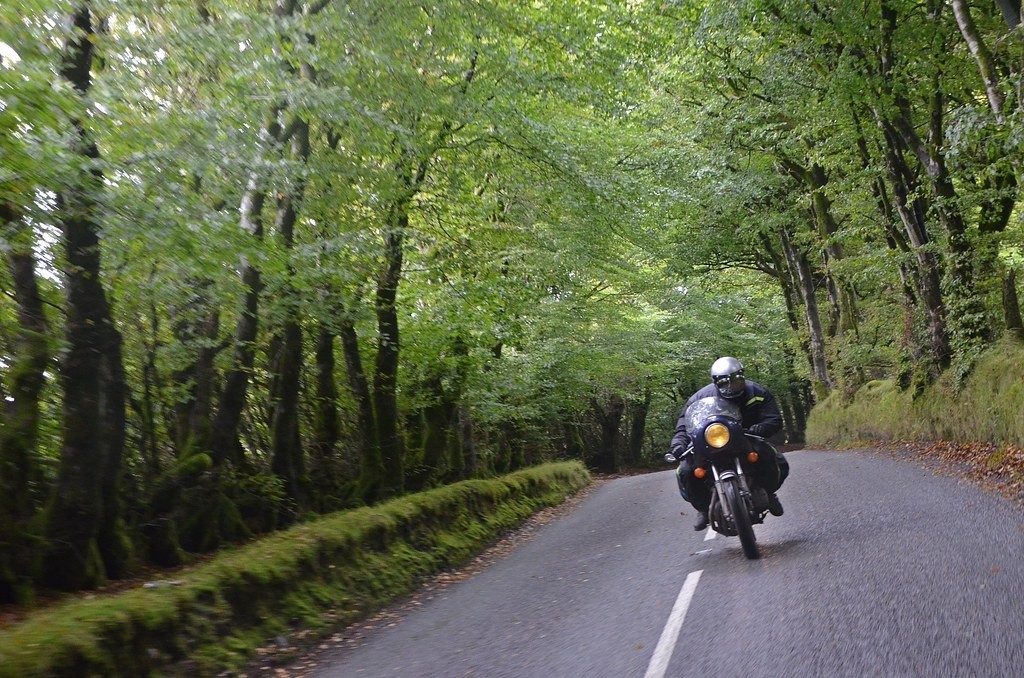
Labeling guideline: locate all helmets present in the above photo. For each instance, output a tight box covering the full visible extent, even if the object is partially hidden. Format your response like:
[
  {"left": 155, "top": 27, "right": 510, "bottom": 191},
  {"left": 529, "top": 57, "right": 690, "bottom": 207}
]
[{"left": 710, "top": 357, "right": 745, "bottom": 398}]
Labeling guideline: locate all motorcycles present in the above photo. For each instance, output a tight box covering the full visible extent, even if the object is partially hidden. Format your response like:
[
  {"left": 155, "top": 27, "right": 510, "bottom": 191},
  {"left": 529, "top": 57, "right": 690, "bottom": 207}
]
[{"left": 663, "top": 397, "right": 766, "bottom": 561}]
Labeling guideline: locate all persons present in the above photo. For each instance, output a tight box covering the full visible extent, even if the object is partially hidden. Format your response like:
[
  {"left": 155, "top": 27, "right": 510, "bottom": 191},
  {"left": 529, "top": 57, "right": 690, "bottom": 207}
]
[{"left": 671, "top": 354, "right": 788, "bottom": 532}]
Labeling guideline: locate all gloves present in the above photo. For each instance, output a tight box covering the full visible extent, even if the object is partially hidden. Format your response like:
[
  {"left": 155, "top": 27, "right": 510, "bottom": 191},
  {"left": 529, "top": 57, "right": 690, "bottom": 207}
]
[
  {"left": 673, "top": 446, "right": 686, "bottom": 460},
  {"left": 749, "top": 424, "right": 766, "bottom": 438}
]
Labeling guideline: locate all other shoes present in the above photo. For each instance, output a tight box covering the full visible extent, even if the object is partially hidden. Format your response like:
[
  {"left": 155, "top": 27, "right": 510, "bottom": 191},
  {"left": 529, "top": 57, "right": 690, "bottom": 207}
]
[
  {"left": 768, "top": 493, "right": 784, "bottom": 516},
  {"left": 693, "top": 510, "right": 710, "bottom": 531}
]
